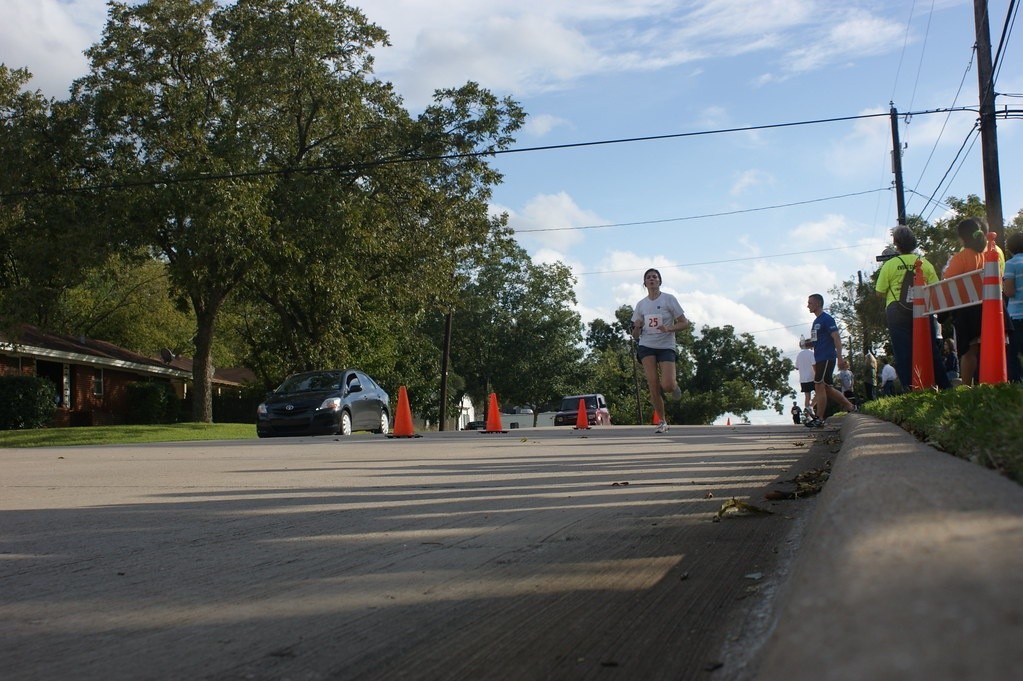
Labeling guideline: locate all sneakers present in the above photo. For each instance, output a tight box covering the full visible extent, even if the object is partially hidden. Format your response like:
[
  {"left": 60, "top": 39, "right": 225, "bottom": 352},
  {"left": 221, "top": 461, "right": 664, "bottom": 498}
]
[
  {"left": 672, "top": 385, "right": 681, "bottom": 401},
  {"left": 655, "top": 419, "right": 669, "bottom": 433}
]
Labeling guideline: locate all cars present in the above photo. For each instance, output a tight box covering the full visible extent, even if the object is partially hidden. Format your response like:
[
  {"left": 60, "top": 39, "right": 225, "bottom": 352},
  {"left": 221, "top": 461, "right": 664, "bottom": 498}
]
[
  {"left": 554, "top": 393, "right": 611, "bottom": 425},
  {"left": 256, "top": 368, "right": 391, "bottom": 437},
  {"left": 461, "top": 421, "right": 486, "bottom": 430}
]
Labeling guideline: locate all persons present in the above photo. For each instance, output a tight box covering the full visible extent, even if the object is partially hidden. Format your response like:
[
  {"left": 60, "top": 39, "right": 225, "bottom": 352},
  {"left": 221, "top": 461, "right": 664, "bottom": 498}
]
[
  {"left": 631, "top": 268, "right": 689, "bottom": 434},
  {"left": 799, "top": 294, "right": 860, "bottom": 427},
  {"left": 874, "top": 216, "right": 1023, "bottom": 394},
  {"left": 791, "top": 337, "right": 897, "bottom": 425}
]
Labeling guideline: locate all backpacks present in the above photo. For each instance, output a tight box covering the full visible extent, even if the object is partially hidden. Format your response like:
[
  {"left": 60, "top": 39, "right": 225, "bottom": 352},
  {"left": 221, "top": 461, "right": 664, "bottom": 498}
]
[{"left": 899, "top": 270, "right": 915, "bottom": 311}]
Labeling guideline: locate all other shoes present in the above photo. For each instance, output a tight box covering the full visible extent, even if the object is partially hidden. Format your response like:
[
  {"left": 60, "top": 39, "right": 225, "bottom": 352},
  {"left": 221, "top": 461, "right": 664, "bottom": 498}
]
[
  {"left": 805, "top": 416, "right": 825, "bottom": 428},
  {"left": 847, "top": 405, "right": 857, "bottom": 415},
  {"left": 804, "top": 416, "right": 813, "bottom": 423},
  {"left": 805, "top": 404, "right": 815, "bottom": 414}
]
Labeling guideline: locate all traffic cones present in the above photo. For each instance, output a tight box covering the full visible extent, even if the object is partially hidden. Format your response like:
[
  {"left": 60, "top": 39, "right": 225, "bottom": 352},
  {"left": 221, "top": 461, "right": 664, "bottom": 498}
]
[
  {"left": 978, "top": 232, "right": 1007, "bottom": 384},
  {"left": 651, "top": 410, "right": 660, "bottom": 425},
  {"left": 573, "top": 399, "right": 592, "bottom": 430},
  {"left": 912, "top": 259, "right": 936, "bottom": 391},
  {"left": 480, "top": 393, "right": 510, "bottom": 434},
  {"left": 727, "top": 418, "right": 730, "bottom": 425},
  {"left": 385, "top": 385, "right": 424, "bottom": 439}
]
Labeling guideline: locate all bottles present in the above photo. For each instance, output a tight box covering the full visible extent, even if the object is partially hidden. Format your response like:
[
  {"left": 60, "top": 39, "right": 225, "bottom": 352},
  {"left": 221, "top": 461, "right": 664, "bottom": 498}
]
[{"left": 800, "top": 334, "right": 805, "bottom": 346}]
